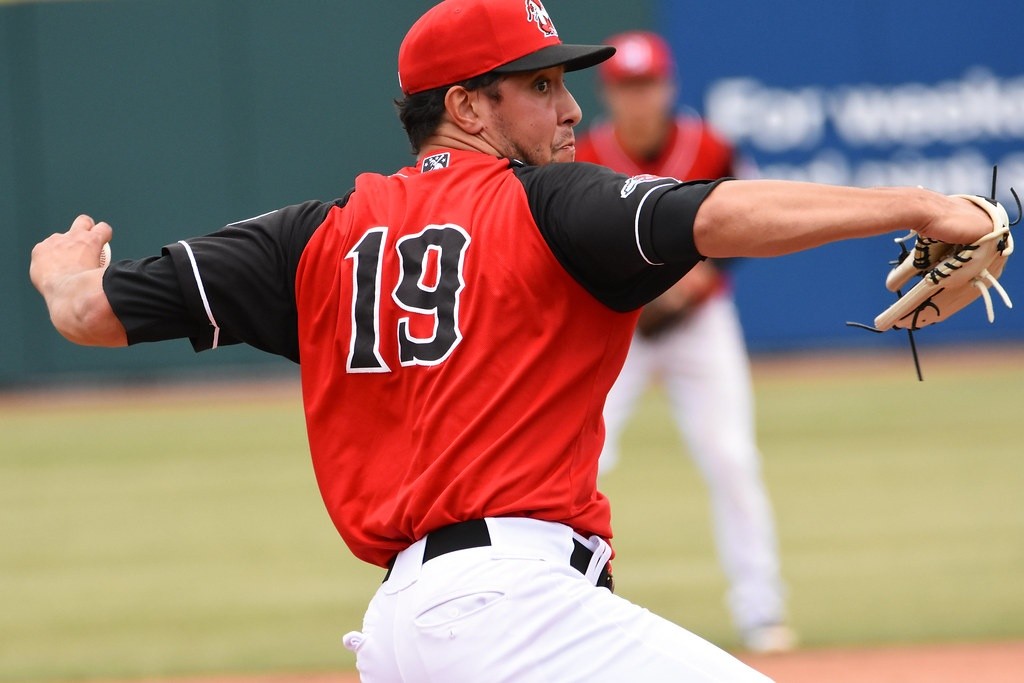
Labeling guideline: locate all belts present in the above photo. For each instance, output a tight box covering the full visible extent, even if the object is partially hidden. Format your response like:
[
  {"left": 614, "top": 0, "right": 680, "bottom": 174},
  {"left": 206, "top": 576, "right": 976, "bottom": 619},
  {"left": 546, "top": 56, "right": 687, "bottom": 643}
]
[{"left": 382, "top": 518, "right": 614, "bottom": 593}]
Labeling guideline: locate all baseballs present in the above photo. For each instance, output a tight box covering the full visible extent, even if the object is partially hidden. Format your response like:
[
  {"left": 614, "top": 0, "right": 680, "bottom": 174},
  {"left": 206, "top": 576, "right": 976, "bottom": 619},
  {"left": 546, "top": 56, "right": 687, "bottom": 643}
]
[{"left": 98, "top": 238, "right": 112, "bottom": 268}]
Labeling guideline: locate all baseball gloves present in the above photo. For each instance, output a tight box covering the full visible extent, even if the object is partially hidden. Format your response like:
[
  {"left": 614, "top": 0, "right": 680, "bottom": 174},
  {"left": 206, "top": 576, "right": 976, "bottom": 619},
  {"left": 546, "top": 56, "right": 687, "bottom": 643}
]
[{"left": 873, "top": 194, "right": 1014, "bottom": 333}]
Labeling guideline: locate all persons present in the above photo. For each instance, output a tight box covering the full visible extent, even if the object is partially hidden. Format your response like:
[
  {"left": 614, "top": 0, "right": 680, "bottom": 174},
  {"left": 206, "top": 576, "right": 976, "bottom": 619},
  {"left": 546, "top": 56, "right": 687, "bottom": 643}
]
[
  {"left": 573, "top": 31, "right": 798, "bottom": 655},
  {"left": 29, "top": 0, "right": 1022, "bottom": 683}
]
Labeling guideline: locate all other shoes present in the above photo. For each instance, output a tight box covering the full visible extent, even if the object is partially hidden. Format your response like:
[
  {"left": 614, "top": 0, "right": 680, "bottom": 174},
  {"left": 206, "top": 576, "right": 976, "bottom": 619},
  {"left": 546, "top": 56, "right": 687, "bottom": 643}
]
[{"left": 745, "top": 624, "right": 796, "bottom": 656}]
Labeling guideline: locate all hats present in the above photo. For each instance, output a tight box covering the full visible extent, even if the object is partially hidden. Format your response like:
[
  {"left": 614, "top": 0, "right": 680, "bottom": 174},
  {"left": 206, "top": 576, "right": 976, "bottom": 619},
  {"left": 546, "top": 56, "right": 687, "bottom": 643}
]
[
  {"left": 599, "top": 31, "right": 671, "bottom": 84},
  {"left": 398, "top": 0, "right": 616, "bottom": 95}
]
